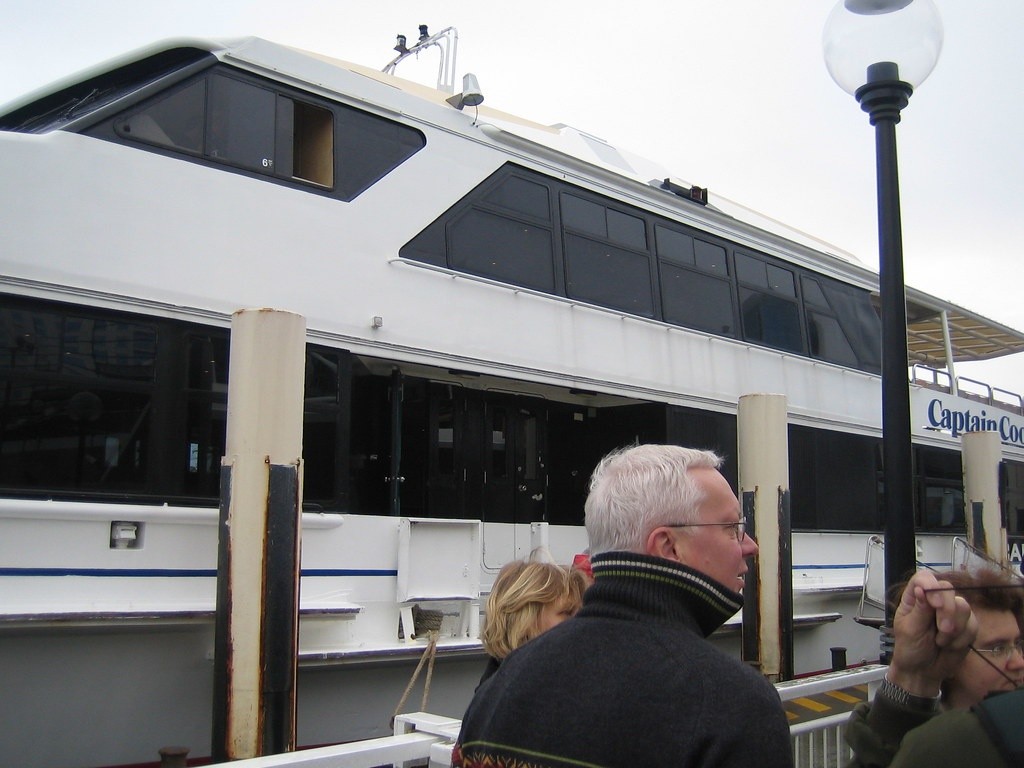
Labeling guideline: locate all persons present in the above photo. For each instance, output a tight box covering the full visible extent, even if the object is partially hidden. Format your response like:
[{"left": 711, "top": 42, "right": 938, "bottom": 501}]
[
  {"left": 845, "top": 565, "right": 1024, "bottom": 768},
  {"left": 460, "top": 445, "right": 796, "bottom": 768},
  {"left": 475, "top": 548, "right": 583, "bottom": 693}
]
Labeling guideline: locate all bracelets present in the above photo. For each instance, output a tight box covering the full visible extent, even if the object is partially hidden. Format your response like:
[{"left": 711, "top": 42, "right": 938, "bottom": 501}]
[{"left": 879, "top": 673, "right": 943, "bottom": 710}]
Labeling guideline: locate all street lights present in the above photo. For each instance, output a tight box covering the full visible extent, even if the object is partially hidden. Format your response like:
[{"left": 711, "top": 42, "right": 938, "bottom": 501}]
[{"left": 820, "top": 0, "right": 943, "bottom": 665}]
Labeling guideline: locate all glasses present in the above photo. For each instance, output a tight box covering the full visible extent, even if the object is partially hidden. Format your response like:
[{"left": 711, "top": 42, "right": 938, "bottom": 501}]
[
  {"left": 970, "top": 640, "right": 1024, "bottom": 661},
  {"left": 648, "top": 516, "right": 747, "bottom": 543}
]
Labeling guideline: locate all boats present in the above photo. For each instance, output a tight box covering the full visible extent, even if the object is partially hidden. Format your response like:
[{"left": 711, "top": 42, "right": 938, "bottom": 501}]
[{"left": 0, "top": 25, "right": 1024, "bottom": 768}]
